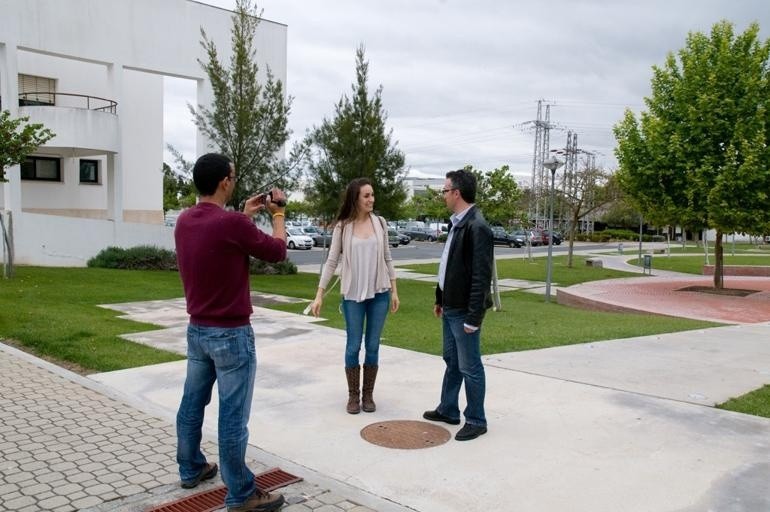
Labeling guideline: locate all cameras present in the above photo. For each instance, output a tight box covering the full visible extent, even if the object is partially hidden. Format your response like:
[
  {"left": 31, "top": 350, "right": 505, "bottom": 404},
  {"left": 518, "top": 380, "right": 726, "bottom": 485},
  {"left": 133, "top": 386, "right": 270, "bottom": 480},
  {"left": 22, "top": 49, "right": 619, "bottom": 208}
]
[{"left": 261, "top": 191, "right": 287, "bottom": 207}]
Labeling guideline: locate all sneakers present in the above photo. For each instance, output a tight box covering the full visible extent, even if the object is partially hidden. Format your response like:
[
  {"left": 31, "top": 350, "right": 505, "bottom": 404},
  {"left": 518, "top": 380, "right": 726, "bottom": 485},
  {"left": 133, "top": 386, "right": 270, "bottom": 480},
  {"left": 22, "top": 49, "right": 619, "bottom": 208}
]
[
  {"left": 226, "top": 487, "right": 285, "bottom": 512},
  {"left": 180, "top": 462, "right": 219, "bottom": 489}
]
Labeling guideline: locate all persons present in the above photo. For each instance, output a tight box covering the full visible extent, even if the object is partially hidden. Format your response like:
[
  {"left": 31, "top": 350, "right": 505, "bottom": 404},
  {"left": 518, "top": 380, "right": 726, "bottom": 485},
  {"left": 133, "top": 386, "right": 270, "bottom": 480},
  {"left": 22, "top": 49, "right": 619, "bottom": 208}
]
[
  {"left": 311, "top": 177, "right": 400, "bottom": 414},
  {"left": 422, "top": 166, "right": 496, "bottom": 442},
  {"left": 171, "top": 151, "right": 291, "bottom": 512}
]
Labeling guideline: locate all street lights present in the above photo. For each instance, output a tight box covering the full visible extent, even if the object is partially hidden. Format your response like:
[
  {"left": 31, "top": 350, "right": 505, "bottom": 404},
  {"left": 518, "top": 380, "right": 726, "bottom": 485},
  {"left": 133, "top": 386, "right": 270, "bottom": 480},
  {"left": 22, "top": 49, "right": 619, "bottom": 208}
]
[{"left": 543, "top": 153, "right": 564, "bottom": 301}]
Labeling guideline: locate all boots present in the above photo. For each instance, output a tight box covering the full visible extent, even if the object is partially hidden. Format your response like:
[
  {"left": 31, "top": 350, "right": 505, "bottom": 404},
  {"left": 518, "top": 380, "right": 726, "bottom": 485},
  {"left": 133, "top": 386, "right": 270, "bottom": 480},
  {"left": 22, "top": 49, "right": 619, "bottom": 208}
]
[
  {"left": 344, "top": 364, "right": 361, "bottom": 414},
  {"left": 361, "top": 364, "right": 379, "bottom": 412}
]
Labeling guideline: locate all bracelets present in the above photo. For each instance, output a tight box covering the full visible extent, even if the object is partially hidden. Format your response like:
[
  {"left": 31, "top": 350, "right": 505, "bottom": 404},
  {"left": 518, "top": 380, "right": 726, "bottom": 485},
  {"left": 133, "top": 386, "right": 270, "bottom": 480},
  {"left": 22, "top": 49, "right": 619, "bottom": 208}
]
[{"left": 270, "top": 211, "right": 285, "bottom": 220}]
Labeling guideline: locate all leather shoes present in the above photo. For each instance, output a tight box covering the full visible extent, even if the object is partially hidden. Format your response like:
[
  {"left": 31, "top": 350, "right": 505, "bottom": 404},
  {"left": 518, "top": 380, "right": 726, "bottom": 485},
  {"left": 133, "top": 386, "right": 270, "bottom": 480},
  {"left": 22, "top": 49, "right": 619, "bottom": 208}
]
[
  {"left": 422, "top": 409, "right": 461, "bottom": 425},
  {"left": 454, "top": 422, "right": 488, "bottom": 440}
]
